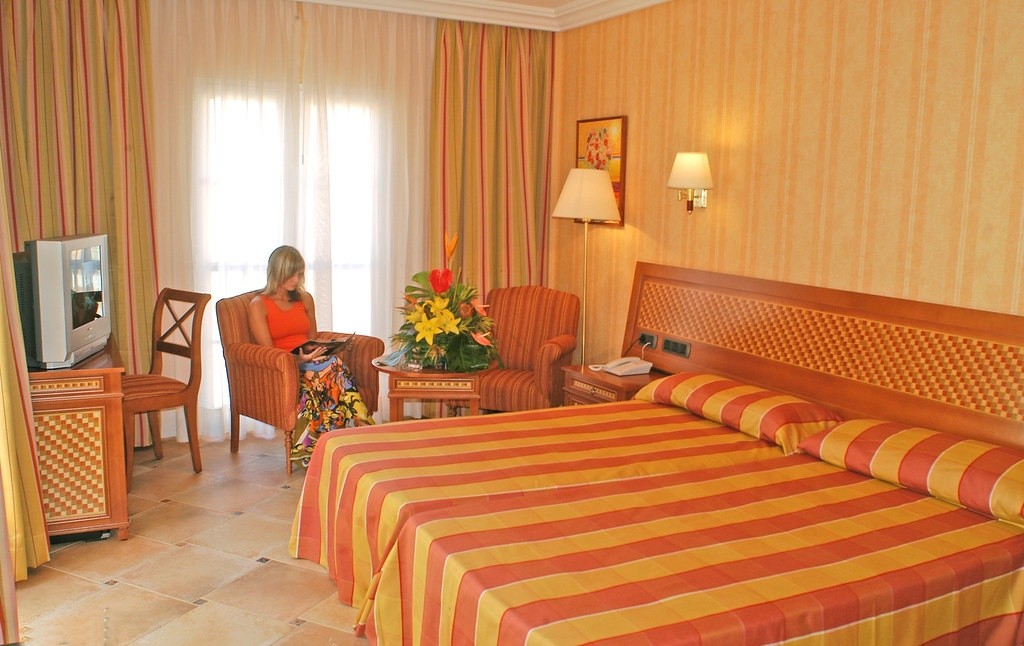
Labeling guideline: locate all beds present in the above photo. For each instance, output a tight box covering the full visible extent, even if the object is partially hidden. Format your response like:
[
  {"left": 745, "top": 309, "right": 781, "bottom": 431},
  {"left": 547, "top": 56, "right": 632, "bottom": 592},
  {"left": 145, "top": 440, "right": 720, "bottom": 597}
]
[{"left": 289, "top": 260, "right": 1024, "bottom": 645}]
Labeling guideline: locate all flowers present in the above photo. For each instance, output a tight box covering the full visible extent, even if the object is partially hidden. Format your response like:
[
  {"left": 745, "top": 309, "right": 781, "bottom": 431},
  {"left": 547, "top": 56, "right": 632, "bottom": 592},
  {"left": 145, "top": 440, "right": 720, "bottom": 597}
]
[{"left": 389, "top": 232, "right": 505, "bottom": 373}]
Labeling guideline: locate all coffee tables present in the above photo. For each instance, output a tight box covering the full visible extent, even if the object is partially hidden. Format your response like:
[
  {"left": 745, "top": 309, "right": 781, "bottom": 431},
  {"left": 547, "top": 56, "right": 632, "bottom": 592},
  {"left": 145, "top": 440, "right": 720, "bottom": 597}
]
[{"left": 371, "top": 353, "right": 498, "bottom": 423}]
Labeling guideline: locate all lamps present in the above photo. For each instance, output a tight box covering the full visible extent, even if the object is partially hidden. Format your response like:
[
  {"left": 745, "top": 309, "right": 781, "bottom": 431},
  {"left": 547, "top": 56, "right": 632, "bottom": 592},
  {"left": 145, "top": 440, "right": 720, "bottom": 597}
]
[
  {"left": 667, "top": 152, "right": 714, "bottom": 212},
  {"left": 551, "top": 169, "right": 621, "bottom": 367}
]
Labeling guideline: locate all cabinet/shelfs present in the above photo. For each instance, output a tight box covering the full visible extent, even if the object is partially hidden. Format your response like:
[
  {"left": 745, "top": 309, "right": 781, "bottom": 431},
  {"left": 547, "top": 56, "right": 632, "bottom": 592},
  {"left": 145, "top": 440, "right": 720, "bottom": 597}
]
[{"left": 29, "top": 331, "right": 130, "bottom": 541}]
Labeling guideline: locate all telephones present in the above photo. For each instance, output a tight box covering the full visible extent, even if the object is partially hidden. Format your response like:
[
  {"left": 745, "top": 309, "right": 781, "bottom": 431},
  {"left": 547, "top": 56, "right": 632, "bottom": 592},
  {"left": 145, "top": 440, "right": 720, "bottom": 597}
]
[{"left": 603, "top": 354, "right": 654, "bottom": 377}]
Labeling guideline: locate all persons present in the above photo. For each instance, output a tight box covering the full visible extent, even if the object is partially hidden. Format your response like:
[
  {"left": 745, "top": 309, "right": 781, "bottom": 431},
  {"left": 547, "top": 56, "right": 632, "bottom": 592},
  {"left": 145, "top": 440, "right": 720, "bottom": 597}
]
[{"left": 248, "top": 245, "right": 377, "bottom": 472}]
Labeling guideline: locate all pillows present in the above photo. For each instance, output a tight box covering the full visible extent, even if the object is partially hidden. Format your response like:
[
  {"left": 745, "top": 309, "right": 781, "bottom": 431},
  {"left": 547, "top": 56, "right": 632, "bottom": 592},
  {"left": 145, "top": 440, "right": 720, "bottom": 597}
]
[
  {"left": 631, "top": 371, "right": 843, "bottom": 456},
  {"left": 795, "top": 417, "right": 1024, "bottom": 527}
]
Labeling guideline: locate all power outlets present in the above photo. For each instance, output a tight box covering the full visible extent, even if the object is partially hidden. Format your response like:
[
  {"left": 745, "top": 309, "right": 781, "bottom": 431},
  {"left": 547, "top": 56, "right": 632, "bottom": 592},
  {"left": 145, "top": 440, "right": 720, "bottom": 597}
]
[
  {"left": 662, "top": 339, "right": 691, "bottom": 358},
  {"left": 639, "top": 333, "right": 658, "bottom": 349}
]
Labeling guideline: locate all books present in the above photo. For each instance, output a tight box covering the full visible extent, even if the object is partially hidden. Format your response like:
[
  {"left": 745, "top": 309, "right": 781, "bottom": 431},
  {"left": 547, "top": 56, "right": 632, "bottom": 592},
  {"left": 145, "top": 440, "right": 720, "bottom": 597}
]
[{"left": 290, "top": 332, "right": 355, "bottom": 358}]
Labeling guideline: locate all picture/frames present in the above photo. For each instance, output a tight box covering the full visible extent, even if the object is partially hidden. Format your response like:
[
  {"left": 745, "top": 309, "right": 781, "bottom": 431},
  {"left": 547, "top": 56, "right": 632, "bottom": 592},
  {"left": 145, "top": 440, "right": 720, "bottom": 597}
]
[{"left": 573, "top": 115, "right": 628, "bottom": 225}]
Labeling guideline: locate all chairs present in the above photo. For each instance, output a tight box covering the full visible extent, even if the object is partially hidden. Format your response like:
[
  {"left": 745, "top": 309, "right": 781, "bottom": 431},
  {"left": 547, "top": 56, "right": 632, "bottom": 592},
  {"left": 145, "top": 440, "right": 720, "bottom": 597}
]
[{"left": 121, "top": 288, "right": 211, "bottom": 493}]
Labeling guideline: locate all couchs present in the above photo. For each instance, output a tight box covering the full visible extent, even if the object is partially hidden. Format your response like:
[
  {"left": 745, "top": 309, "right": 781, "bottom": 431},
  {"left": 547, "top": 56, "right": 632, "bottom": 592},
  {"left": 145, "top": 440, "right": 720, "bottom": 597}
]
[
  {"left": 458, "top": 284, "right": 579, "bottom": 412},
  {"left": 217, "top": 288, "right": 384, "bottom": 471}
]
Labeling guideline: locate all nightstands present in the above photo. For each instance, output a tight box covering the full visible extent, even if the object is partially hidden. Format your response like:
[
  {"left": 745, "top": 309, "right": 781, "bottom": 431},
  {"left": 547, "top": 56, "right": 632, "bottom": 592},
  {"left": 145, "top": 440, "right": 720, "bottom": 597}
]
[{"left": 560, "top": 360, "right": 666, "bottom": 404}]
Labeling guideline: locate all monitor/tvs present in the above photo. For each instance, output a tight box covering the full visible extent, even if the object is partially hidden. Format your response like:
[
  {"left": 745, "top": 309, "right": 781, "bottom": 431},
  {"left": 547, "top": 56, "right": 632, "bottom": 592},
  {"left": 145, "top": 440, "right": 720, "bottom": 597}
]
[{"left": 12, "top": 233, "right": 112, "bottom": 369}]
